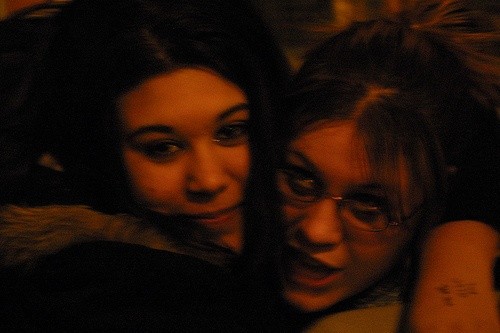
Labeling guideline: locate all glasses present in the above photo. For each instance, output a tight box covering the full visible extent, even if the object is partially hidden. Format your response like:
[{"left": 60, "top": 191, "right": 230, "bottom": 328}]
[{"left": 273, "top": 165, "right": 424, "bottom": 232}]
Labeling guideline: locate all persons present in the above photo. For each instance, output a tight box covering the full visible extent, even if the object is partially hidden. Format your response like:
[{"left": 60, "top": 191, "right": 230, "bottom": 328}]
[
  {"left": 262, "top": 0, "right": 500, "bottom": 332},
  {"left": 0, "top": 1, "right": 500, "bottom": 332}
]
